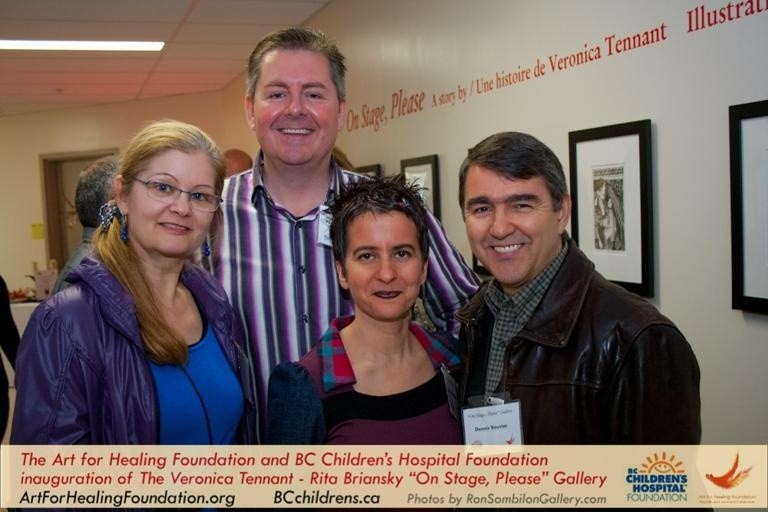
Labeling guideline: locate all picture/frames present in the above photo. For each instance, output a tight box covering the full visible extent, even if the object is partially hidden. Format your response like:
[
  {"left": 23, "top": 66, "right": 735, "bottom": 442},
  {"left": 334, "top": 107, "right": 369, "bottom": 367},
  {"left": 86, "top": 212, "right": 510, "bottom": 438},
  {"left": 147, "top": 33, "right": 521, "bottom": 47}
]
[{"left": 567, "top": 98, "right": 768, "bottom": 311}]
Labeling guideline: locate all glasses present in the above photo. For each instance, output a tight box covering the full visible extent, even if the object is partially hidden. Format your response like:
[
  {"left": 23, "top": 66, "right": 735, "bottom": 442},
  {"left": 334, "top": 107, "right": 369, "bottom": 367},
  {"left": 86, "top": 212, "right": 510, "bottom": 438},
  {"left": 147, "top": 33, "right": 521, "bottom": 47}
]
[{"left": 131, "top": 176, "right": 223, "bottom": 213}]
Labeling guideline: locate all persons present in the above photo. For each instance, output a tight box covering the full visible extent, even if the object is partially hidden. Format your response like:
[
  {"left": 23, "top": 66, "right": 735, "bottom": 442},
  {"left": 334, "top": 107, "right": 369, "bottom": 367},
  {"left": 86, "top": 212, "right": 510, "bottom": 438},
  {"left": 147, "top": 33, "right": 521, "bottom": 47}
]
[{"left": 0, "top": 26, "right": 701, "bottom": 445}]
